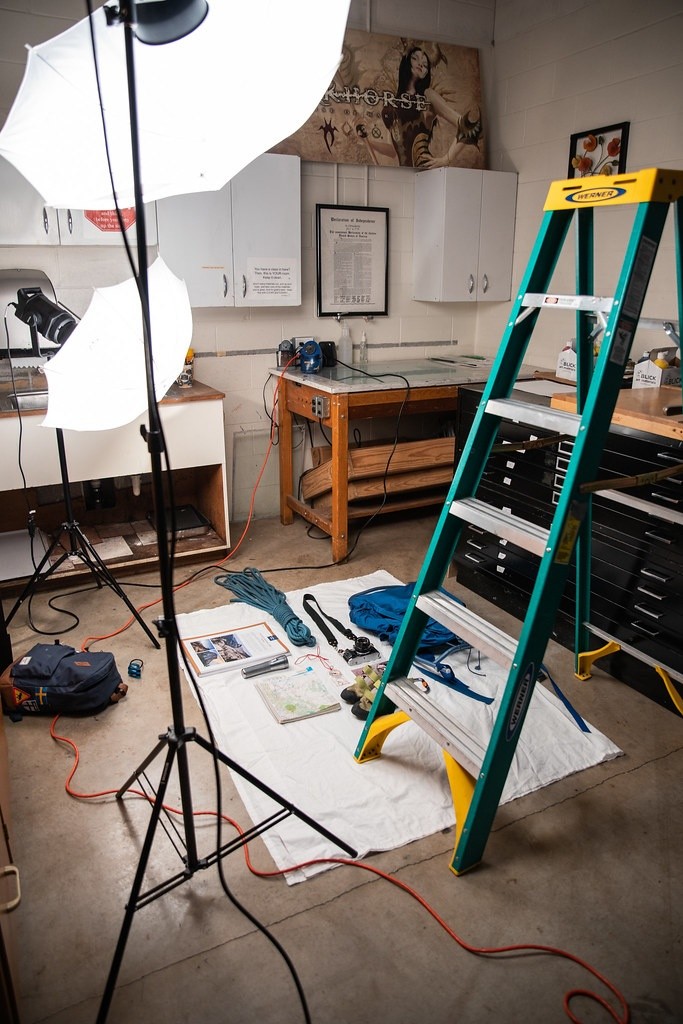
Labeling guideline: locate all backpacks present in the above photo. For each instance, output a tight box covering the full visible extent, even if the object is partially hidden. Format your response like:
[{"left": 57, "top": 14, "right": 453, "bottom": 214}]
[
  {"left": 347, "top": 582, "right": 472, "bottom": 665},
  {"left": 0, "top": 643, "right": 128, "bottom": 717}
]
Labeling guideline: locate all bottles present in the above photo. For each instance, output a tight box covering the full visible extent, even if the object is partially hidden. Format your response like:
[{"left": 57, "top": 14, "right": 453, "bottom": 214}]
[
  {"left": 562, "top": 342, "right": 571, "bottom": 352},
  {"left": 338, "top": 319, "right": 353, "bottom": 365},
  {"left": 637, "top": 352, "right": 650, "bottom": 365},
  {"left": 653, "top": 352, "right": 669, "bottom": 369}
]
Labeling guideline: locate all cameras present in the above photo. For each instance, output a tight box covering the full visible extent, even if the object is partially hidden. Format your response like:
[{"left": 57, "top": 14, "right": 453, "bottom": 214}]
[{"left": 343, "top": 636, "right": 381, "bottom": 666}]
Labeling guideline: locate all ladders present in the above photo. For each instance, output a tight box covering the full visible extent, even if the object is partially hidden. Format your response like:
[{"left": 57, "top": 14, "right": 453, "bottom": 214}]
[{"left": 354, "top": 165, "right": 683, "bottom": 876}]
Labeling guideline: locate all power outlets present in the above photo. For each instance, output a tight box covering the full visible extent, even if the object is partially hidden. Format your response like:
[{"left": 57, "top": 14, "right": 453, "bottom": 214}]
[{"left": 294, "top": 337, "right": 314, "bottom": 350}]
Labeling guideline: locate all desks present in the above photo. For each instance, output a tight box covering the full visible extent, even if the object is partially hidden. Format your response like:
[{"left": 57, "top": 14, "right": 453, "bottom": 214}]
[{"left": 268, "top": 355, "right": 556, "bottom": 563}]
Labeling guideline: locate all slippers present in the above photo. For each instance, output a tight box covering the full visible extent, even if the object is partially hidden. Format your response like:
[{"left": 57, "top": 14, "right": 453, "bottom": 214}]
[
  {"left": 352, "top": 678, "right": 430, "bottom": 718},
  {"left": 341, "top": 660, "right": 391, "bottom": 704}
]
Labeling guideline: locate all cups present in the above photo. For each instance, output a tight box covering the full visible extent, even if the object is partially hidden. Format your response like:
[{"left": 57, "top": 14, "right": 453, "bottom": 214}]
[{"left": 176, "top": 364, "right": 193, "bottom": 388}]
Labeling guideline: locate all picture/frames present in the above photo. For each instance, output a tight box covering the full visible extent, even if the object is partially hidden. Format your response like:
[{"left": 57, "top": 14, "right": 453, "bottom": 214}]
[{"left": 567, "top": 122, "right": 630, "bottom": 178}]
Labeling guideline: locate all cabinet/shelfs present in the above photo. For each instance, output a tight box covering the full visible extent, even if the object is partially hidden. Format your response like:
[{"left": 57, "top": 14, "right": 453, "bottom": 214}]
[
  {"left": 0, "top": 155, "right": 158, "bottom": 247},
  {"left": 413, "top": 167, "right": 517, "bottom": 302},
  {"left": 455, "top": 380, "right": 683, "bottom": 717},
  {"left": 156, "top": 153, "right": 301, "bottom": 309}
]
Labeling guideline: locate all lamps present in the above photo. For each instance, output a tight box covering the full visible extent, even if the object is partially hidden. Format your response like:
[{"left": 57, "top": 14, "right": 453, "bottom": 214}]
[
  {"left": 6, "top": 256, "right": 192, "bottom": 649},
  {"left": 0, "top": 0, "right": 352, "bottom": 1024}
]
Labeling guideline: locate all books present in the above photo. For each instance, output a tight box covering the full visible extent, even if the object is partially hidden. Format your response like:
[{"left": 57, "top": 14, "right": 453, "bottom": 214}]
[
  {"left": 252, "top": 666, "right": 341, "bottom": 723},
  {"left": 181, "top": 622, "right": 291, "bottom": 677}
]
